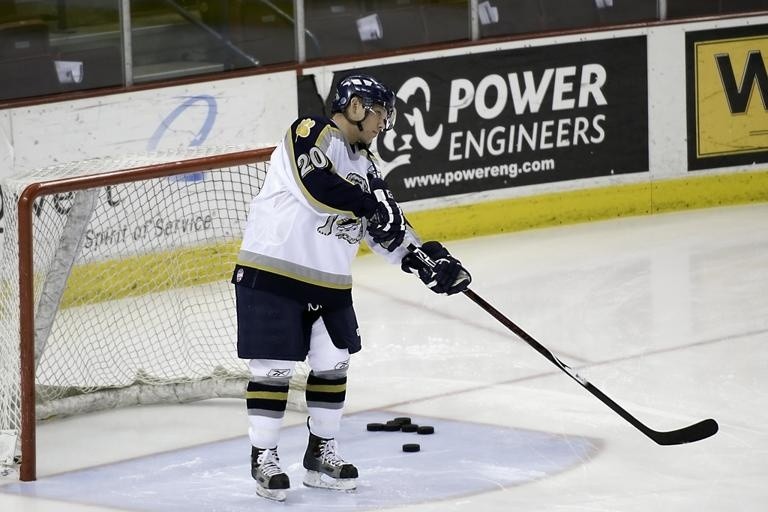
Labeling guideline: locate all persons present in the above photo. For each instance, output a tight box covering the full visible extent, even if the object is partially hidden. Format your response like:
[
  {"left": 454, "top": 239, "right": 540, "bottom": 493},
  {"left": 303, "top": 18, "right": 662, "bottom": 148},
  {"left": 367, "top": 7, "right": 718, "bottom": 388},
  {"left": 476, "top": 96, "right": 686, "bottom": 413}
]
[{"left": 229, "top": 73, "right": 473, "bottom": 501}]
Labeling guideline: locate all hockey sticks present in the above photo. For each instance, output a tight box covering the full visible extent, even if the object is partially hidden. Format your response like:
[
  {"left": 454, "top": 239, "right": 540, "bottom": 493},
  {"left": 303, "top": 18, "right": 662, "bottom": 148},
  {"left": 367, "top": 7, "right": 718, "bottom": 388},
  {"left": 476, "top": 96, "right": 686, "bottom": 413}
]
[{"left": 401, "top": 238, "right": 717, "bottom": 446}]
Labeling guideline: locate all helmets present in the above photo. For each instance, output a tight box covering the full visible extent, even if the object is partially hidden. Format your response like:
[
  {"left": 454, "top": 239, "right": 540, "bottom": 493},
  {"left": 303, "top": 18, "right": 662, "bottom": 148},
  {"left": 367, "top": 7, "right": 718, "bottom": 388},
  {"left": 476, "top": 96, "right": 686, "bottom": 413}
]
[{"left": 331, "top": 74, "right": 397, "bottom": 130}]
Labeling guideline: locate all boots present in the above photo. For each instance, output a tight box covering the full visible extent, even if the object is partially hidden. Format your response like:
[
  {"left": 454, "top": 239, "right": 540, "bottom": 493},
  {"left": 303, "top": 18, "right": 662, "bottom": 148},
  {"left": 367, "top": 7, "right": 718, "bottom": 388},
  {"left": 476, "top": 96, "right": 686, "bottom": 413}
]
[
  {"left": 302, "top": 430, "right": 359, "bottom": 478},
  {"left": 250, "top": 446, "right": 290, "bottom": 490}
]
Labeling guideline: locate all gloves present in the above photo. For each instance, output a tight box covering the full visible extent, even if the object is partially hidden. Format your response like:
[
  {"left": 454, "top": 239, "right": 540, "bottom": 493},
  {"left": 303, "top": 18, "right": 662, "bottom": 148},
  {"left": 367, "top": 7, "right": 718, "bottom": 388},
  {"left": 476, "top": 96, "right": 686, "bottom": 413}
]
[
  {"left": 365, "top": 198, "right": 407, "bottom": 253},
  {"left": 401, "top": 241, "right": 472, "bottom": 297}
]
